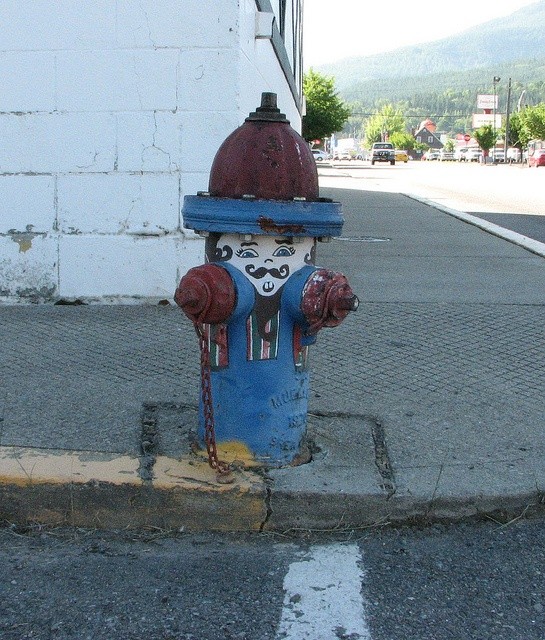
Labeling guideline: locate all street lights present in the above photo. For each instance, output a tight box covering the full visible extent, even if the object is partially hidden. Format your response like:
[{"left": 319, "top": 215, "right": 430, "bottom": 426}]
[{"left": 493, "top": 76, "right": 500, "bottom": 164}]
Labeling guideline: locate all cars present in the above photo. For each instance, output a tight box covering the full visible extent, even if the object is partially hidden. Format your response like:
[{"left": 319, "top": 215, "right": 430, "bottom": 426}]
[
  {"left": 465, "top": 148, "right": 482, "bottom": 162},
  {"left": 426, "top": 149, "right": 441, "bottom": 161},
  {"left": 454, "top": 148, "right": 467, "bottom": 161},
  {"left": 489, "top": 149, "right": 504, "bottom": 162},
  {"left": 311, "top": 151, "right": 327, "bottom": 161},
  {"left": 395, "top": 151, "right": 408, "bottom": 163},
  {"left": 339, "top": 152, "right": 351, "bottom": 161},
  {"left": 439, "top": 148, "right": 453, "bottom": 161},
  {"left": 529, "top": 149, "right": 545, "bottom": 167},
  {"left": 334, "top": 154, "right": 338, "bottom": 161}
]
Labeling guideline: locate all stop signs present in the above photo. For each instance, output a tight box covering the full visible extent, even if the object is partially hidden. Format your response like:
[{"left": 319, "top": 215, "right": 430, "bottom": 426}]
[{"left": 464, "top": 135, "right": 470, "bottom": 141}]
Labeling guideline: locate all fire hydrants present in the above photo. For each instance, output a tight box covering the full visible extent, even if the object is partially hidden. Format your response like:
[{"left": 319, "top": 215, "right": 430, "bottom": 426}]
[{"left": 174, "top": 92, "right": 359, "bottom": 472}]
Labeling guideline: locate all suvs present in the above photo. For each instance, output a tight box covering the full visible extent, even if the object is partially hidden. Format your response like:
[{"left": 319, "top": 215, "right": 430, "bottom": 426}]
[{"left": 369, "top": 143, "right": 395, "bottom": 165}]
